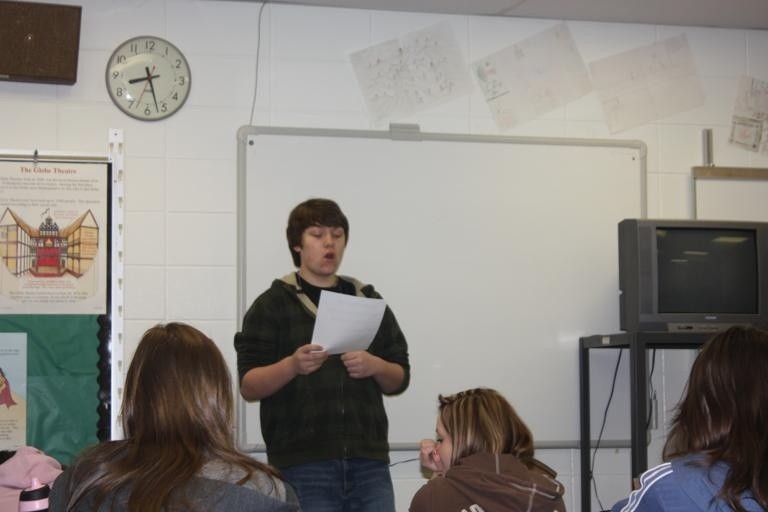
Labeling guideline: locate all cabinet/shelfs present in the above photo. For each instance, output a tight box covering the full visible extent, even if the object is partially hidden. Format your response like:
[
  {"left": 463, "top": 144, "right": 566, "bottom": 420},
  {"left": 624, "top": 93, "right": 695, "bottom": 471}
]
[{"left": 578, "top": 330, "right": 723, "bottom": 512}]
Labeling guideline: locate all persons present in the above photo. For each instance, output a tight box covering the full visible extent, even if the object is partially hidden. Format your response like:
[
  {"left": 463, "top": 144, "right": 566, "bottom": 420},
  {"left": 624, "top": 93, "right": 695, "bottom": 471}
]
[
  {"left": 47, "top": 321, "right": 303, "bottom": 511},
  {"left": 233, "top": 198, "right": 411, "bottom": 512},
  {"left": 407, "top": 386, "right": 567, "bottom": 512},
  {"left": 609, "top": 322, "right": 768, "bottom": 511}
]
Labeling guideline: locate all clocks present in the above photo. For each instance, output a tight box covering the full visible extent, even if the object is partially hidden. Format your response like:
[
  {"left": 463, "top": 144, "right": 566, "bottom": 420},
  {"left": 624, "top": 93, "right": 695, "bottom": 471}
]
[{"left": 105, "top": 34, "right": 191, "bottom": 121}]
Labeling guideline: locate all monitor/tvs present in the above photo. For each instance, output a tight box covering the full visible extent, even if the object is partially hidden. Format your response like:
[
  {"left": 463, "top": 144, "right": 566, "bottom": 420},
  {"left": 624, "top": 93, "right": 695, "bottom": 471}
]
[{"left": 618, "top": 218, "right": 768, "bottom": 333}]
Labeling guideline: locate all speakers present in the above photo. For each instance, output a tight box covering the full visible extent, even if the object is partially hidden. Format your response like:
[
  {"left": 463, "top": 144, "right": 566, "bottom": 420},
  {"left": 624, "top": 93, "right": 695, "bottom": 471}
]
[{"left": 0, "top": 1, "right": 83, "bottom": 85}]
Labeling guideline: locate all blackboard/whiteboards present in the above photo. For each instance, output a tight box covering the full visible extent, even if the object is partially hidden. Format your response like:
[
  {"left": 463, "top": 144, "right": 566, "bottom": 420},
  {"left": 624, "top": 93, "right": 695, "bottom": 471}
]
[{"left": 236, "top": 125, "right": 649, "bottom": 454}]
